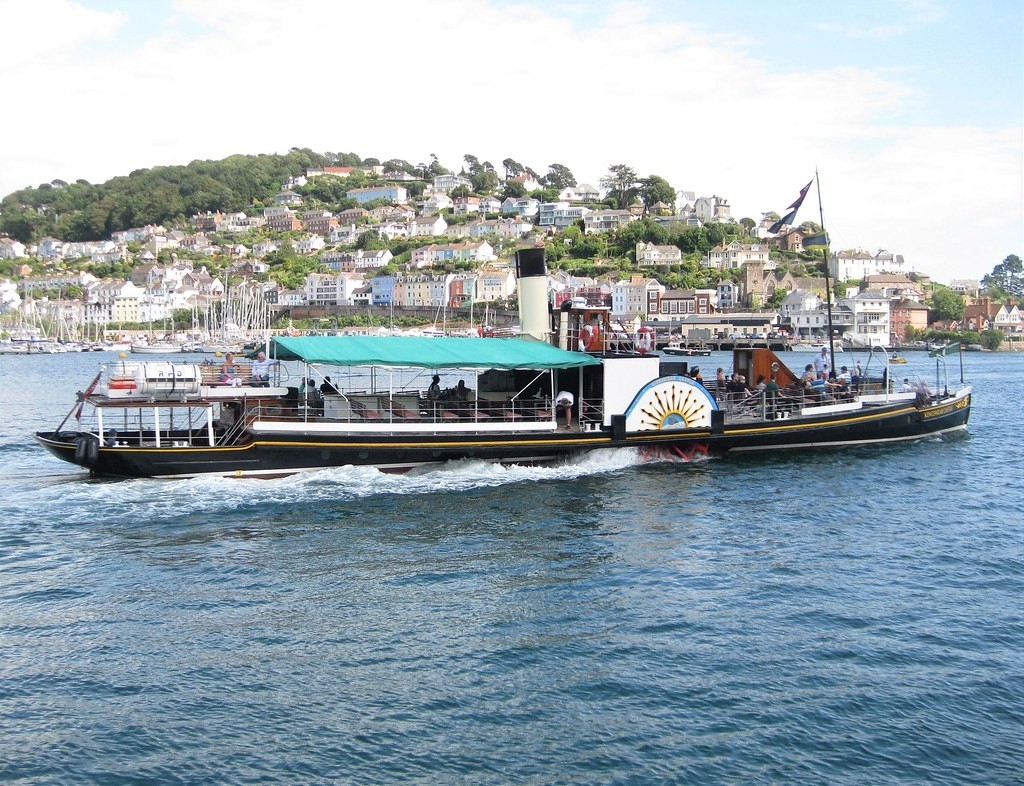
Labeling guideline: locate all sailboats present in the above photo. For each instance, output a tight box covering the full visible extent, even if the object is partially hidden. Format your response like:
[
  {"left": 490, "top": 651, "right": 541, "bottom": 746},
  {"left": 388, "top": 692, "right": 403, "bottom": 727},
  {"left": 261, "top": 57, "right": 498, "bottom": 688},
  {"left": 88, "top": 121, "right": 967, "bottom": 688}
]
[
  {"left": 0, "top": 271, "right": 267, "bottom": 354},
  {"left": 791, "top": 282, "right": 844, "bottom": 353}
]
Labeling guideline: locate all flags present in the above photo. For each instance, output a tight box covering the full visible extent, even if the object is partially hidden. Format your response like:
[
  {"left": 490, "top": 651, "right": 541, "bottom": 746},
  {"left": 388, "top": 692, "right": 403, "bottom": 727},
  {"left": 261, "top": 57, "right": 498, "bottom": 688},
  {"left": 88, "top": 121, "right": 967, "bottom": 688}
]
[
  {"left": 769, "top": 182, "right": 812, "bottom": 233},
  {"left": 802, "top": 230, "right": 826, "bottom": 251}
]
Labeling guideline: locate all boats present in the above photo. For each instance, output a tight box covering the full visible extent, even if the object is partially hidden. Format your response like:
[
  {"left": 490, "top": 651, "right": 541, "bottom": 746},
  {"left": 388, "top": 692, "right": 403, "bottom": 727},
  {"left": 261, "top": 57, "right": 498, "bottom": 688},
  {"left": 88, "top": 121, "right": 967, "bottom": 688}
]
[
  {"left": 31, "top": 247, "right": 971, "bottom": 481},
  {"left": 889, "top": 357, "right": 907, "bottom": 364},
  {"left": 662, "top": 336, "right": 712, "bottom": 356},
  {"left": 423, "top": 326, "right": 444, "bottom": 337}
]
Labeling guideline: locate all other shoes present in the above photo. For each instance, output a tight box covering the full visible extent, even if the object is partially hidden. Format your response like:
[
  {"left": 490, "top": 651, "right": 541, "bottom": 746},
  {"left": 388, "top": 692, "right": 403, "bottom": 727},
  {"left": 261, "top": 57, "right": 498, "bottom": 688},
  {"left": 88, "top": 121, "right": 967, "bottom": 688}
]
[{"left": 566, "top": 425, "right": 570, "bottom": 429}]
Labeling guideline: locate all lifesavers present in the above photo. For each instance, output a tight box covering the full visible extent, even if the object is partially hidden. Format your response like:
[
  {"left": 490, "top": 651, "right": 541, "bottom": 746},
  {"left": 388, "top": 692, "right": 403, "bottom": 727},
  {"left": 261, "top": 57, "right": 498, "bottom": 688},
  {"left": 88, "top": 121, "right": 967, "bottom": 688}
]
[
  {"left": 635, "top": 329, "right": 653, "bottom": 354},
  {"left": 578, "top": 325, "right": 595, "bottom": 353}
]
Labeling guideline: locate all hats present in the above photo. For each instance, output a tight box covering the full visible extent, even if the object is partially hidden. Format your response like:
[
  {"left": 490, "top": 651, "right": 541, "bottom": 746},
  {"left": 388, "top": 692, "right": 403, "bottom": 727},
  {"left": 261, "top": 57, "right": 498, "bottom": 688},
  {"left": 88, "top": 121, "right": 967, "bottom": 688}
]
[{"left": 562, "top": 398, "right": 568, "bottom": 404}]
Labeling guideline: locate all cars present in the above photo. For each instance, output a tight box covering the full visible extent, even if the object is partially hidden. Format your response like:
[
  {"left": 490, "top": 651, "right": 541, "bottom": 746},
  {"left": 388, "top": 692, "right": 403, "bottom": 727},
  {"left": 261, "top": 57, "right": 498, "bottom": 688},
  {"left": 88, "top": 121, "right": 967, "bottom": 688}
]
[{"left": 714, "top": 331, "right": 780, "bottom": 339}]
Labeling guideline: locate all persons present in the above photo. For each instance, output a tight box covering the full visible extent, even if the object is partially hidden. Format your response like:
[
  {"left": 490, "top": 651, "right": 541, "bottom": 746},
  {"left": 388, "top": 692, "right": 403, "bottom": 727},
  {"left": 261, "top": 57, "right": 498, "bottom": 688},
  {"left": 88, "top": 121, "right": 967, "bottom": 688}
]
[
  {"left": 108, "top": 430, "right": 117, "bottom": 445},
  {"left": 320, "top": 376, "right": 337, "bottom": 392},
  {"left": 298, "top": 377, "right": 320, "bottom": 416},
  {"left": 548, "top": 391, "right": 573, "bottom": 430},
  {"left": 249, "top": 352, "right": 280, "bottom": 387},
  {"left": 716, "top": 368, "right": 783, "bottom": 416},
  {"left": 428, "top": 375, "right": 440, "bottom": 398},
  {"left": 220, "top": 353, "right": 242, "bottom": 388},
  {"left": 800, "top": 347, "right": 861, "bottom": 401},
  {"left": 900, "top": 379, "right": 931, "bottom": 400},
  {"left": 458, "top": 380, "right": 470, "bottom": 398}
]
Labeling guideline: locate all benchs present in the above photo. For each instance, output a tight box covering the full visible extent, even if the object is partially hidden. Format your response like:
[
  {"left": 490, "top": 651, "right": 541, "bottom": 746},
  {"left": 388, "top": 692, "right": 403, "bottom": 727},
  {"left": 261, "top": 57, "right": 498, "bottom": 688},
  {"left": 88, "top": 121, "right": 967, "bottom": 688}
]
[
  {"left": 700, "top": 379, "right": 857, "bottom": 413},
  {"left": 424, "top": 399, "right": 459, "bottom": 423},
  {"left": 519, "top": 408, "right": 552, "bottom": 421},
  {"left": 350, "top": 398, "right": 378, "bottom": 419},
  {"left": 197, "top": 364, "right": 270, "bottom": 388},
  {"left": 458, "top": 404, "right": 490, "bottom": 423},
  {"left": 381, "top": 397, "right": 421, "bottom": 423},
  {"left": 487, "top": 406, "right": 523, "bottom": 422},
  {"left": 298, "top": 392, "right": 324, "bottom": 415}
]
